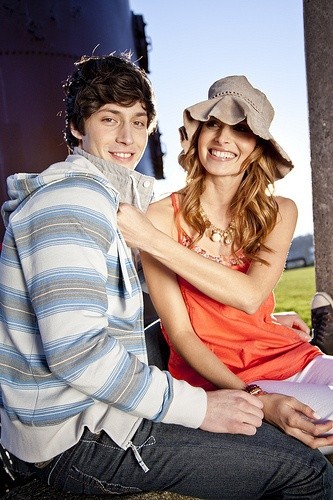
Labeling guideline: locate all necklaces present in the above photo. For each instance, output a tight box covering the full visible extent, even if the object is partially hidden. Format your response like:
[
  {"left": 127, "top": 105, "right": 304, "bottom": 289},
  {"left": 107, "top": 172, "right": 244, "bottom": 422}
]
[{"left": 197, "top": 204, "right": 240, "bottom": 245}]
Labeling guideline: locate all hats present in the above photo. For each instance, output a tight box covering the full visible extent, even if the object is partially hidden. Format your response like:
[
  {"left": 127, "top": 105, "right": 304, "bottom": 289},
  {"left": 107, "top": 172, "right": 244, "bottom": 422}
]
[{"left": 177, "top": 74, "right": 294, "bottom": 184}]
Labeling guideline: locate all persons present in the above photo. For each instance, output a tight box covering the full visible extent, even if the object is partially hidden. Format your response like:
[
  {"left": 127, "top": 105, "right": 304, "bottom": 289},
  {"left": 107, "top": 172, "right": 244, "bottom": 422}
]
[
  {"left": 1, "top": 53, "right": 333, "bottom": 500},
  {"left": 115, "top": 75, "right": 333, "bottom": 456}
]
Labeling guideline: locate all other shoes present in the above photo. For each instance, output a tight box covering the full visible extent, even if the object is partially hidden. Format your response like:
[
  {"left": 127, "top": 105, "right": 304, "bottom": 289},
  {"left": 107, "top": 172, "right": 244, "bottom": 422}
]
[{"left": 309, "top": 291, "right": 332, "bottom": 355}]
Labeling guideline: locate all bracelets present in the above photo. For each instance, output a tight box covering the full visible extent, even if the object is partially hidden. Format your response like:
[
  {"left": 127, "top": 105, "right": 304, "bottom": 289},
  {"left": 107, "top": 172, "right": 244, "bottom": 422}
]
[{"left": 242, "top": 383, "right": 268, "bottom": 397}]
